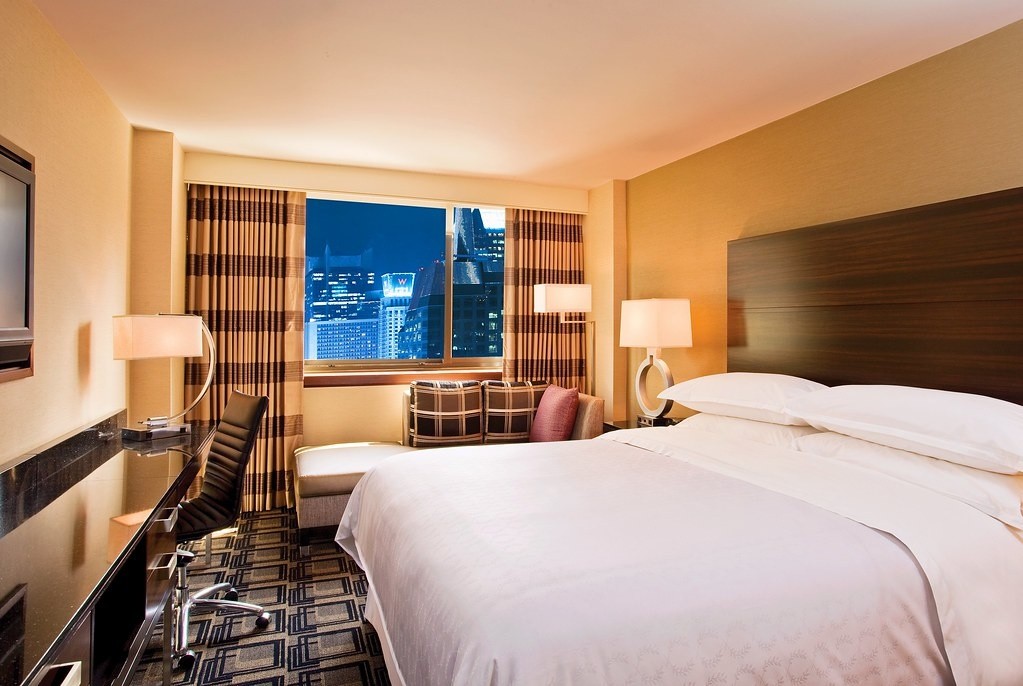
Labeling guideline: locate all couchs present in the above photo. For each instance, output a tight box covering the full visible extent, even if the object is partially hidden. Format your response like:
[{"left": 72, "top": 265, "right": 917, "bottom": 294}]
[{"left": 291, "top": 394, "right": 600, "bottom": 546}]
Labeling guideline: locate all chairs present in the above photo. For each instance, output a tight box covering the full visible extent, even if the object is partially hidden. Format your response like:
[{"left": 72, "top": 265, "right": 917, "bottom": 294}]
[{"left": 173, "top": 389, "right": 270, "bottom": 668}]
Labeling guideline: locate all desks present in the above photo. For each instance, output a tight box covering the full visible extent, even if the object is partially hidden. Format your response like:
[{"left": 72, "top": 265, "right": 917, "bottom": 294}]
[{"left": 0, "top": 414, "right": 219, "bottom": 686}]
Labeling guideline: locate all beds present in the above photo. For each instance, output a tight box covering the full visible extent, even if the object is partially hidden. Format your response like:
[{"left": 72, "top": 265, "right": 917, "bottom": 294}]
[{"left": 334, "top": 187, "right": 1023, "bottom": 686}]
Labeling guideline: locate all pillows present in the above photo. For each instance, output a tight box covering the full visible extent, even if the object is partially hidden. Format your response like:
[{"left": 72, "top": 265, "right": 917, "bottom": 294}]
[
  {"left": 798, "top": 384, "right": 1023, "bottom": 476},
  {"left": 671, "top": 412, "right": 825, "bottom": 449},
  {"left": 529, "top": 383, "right": 577, "bottom": 444},
  {"left": 410, "top": 380, "right": 484, "bottom": 449},
  {"left": 796, "top": 429, "right": 1023, "bottom": 531},
  {"left": 657, "top": 371, "right": 831, "bottom": 426},
  {"left": 483, "top": 377, "right": 550, "bottom": 442}
]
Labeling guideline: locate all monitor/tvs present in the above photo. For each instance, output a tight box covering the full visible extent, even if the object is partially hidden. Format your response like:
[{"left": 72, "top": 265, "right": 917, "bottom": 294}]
[{"left": 0, "top": 154, "right": 36, "bottom": 365}]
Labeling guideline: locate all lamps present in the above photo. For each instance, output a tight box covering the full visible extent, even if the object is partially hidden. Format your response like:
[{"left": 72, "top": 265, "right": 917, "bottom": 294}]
[
  {"left": 112, "top": 311, "right": 217, "bottom": 441},
  {"left": 620, "top": 299, "right": 692, "bottom": 426},
  {"left": 533, "top": 284, "right": 596, "bottom": 397}
]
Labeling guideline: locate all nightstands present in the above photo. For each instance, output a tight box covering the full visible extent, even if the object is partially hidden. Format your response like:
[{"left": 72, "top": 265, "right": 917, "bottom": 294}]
[{"left": 603, "top": 419, "right": 646, "bottom": 433}]
